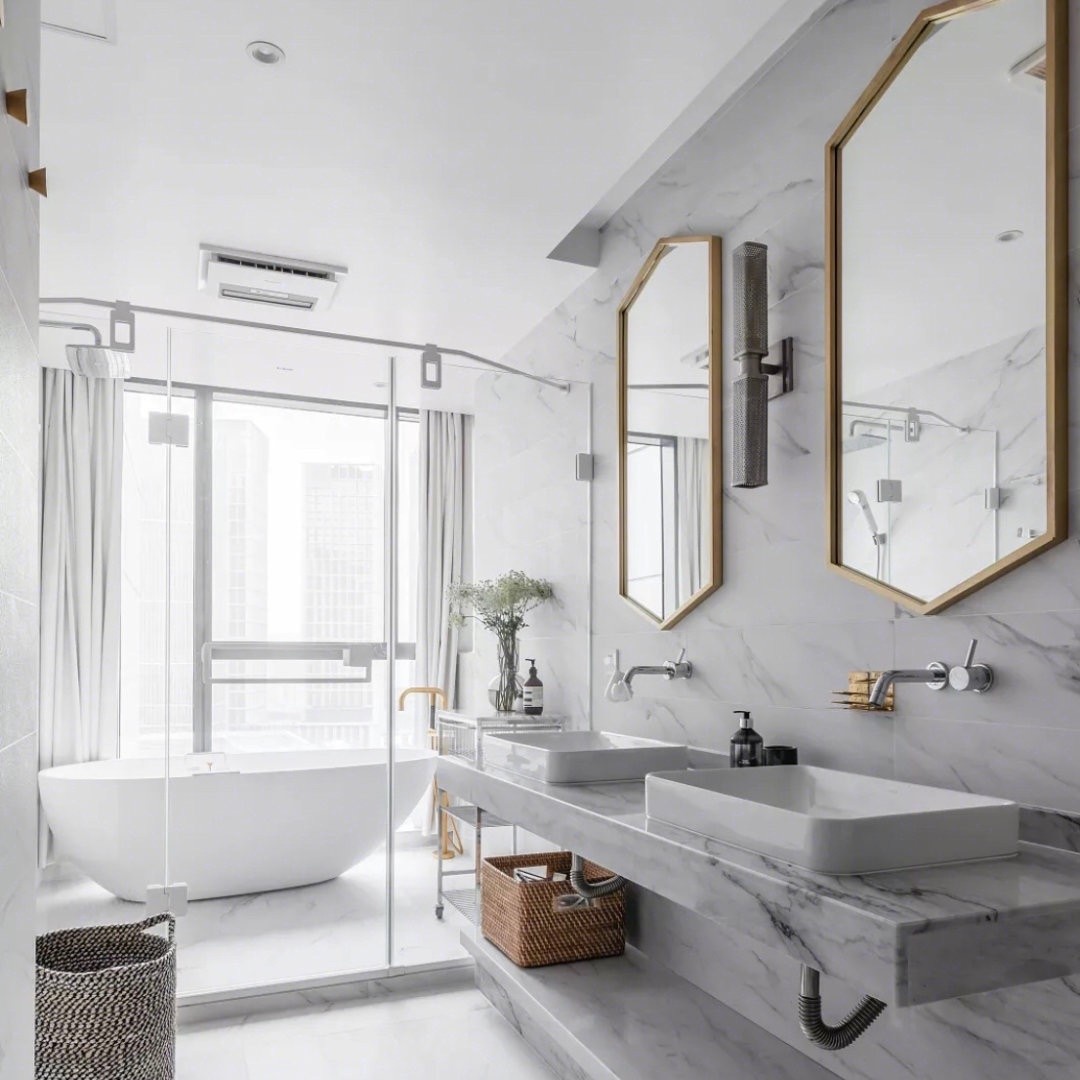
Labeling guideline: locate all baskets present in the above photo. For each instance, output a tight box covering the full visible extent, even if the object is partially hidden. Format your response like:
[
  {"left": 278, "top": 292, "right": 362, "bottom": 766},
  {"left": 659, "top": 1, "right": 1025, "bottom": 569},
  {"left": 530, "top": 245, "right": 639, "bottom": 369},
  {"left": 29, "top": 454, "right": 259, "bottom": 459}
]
[
  {"left": 34, "top": 912, "right": 176, "bottom": 1080},
  {"left": 481, "top": 851, "right": 626, "bottom": 968}
]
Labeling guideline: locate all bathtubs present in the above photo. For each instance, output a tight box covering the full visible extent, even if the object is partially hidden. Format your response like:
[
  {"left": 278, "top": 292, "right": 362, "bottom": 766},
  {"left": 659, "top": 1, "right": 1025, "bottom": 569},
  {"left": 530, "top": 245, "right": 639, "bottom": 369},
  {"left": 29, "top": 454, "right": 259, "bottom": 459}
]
[{"left": 37, "top": 746, "right": 439, "bottom": 902}]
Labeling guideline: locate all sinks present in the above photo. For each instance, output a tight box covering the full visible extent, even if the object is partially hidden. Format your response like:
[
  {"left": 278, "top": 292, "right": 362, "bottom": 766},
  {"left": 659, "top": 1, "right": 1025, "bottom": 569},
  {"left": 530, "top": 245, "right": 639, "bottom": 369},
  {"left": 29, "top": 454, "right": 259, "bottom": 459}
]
[
  {"left": 643, "top": 762, "right": 1023, "bottom": 879},
  {"left": 480, "top": 728, "right": 690, "bottom": 785}
]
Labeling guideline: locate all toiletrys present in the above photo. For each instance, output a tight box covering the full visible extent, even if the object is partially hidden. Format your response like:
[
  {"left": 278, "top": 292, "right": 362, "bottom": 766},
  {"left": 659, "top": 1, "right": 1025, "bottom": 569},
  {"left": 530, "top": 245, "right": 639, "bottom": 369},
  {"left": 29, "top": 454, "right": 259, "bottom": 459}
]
[
  {"left": 522, "top": 658, "right": 544, "bottom": 715},
  {"left": 729, "top": 710, "right": 763, "bottom": 768}
]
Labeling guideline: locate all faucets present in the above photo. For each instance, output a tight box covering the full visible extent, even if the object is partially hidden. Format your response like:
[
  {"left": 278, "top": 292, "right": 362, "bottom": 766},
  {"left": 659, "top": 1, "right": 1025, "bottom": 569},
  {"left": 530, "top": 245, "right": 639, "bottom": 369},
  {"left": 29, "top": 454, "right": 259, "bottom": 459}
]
[
  {"left": 867, "top": 661, "right": 951, "bottom": 709},
  {"left": 396, "top": 687, "right": 448, "bottom": 712},
  {"left": 613, "top": 648, "right": 692, "bottom": 700}
]
